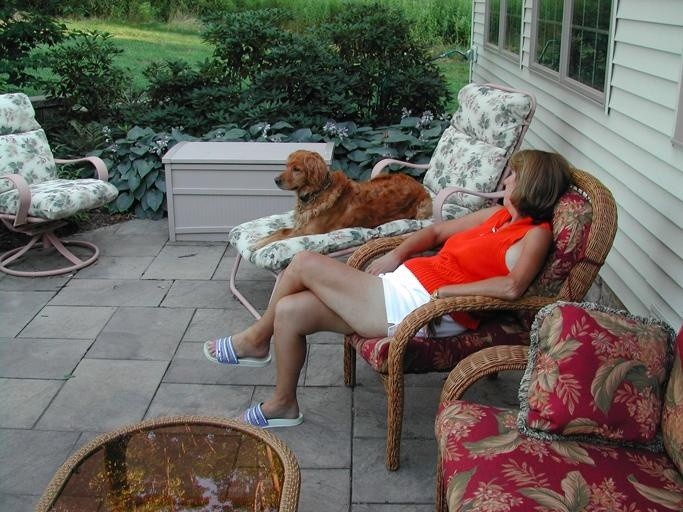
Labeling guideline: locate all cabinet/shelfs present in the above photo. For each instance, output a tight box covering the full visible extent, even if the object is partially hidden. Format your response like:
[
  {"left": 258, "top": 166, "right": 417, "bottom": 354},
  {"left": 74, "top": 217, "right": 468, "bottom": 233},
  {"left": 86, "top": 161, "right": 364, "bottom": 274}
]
[{"left": 162, "top": 140, "right": 334, "bottom": 246}]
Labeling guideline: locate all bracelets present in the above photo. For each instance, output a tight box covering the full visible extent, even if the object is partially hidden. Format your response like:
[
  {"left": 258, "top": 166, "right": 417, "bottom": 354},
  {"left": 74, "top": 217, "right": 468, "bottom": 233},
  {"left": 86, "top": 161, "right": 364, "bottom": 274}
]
[{"left": 429, "top": 286, "right": 439, "bottom": 301}]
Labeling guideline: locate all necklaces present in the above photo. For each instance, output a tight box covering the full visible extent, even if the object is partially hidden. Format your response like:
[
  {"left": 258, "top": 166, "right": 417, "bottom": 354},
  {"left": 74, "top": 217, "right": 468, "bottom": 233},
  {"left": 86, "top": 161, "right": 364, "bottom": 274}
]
[{"left": 491, "top": 214, "right": 509, "bottom": 233}]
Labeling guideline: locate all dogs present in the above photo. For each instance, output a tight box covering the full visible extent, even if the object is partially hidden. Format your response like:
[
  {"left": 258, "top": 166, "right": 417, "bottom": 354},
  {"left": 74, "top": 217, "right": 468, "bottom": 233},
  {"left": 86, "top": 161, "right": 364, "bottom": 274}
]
[{"left": 248, "top": 149, "right": 434, "bottom": 253}]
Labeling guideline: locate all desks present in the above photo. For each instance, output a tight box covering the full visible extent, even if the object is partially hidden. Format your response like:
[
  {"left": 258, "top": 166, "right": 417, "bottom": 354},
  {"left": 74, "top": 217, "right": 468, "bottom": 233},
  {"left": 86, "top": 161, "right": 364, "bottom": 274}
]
[{"left": 31, "top": 414, "right": 300, "bottom": 512}]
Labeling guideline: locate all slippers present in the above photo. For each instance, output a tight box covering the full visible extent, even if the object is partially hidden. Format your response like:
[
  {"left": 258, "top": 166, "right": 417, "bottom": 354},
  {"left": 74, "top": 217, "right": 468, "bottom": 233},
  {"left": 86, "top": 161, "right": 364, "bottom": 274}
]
[
  {"left": 235, "top": 402, "right": 304, "bottom": 428},
  {"left": 203, "top": 335, "right": 271, "bottom": 368}
]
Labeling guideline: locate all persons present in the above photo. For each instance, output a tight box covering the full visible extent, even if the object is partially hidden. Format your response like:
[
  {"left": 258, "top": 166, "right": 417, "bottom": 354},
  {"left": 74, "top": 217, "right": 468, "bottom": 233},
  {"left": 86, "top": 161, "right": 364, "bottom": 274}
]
[{"left": 202, "top": 148, "right": 571, "bottom": 433}]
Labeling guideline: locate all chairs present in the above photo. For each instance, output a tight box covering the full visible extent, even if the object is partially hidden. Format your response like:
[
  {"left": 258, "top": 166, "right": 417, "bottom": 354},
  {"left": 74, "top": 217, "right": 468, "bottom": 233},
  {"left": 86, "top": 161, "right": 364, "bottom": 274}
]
[
  {"left": 0, "top": 92, "right": 119, "bottom": 276},
  {"left": 430, "top": 313, "right": 683, "bottom": 512}
]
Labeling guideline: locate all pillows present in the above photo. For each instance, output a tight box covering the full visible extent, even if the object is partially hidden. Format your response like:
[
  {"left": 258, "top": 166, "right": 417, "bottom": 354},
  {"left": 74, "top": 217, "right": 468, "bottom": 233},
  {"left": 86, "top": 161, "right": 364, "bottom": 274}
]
[{"left": 519, "top": 303, "right": 673, "bottom": 451}]
